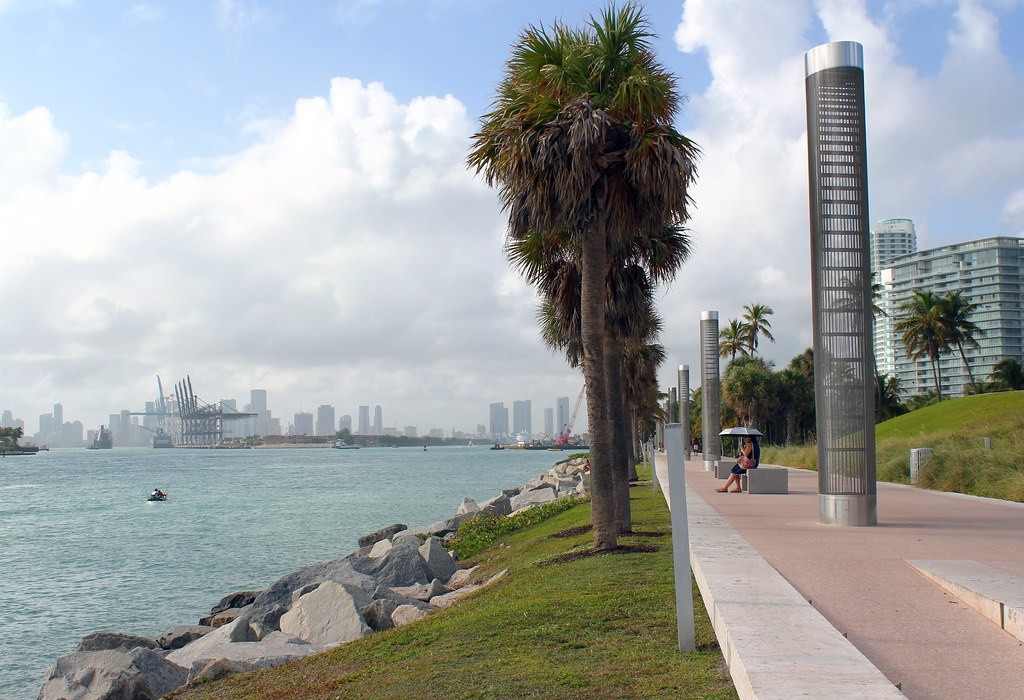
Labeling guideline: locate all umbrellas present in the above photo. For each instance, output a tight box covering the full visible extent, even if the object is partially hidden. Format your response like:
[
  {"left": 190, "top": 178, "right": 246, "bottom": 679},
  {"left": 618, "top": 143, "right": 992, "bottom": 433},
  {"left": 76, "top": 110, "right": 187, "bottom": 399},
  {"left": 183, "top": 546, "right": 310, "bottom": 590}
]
[{"left": 718, "top": 427, "right": 763, "bottom": 443}]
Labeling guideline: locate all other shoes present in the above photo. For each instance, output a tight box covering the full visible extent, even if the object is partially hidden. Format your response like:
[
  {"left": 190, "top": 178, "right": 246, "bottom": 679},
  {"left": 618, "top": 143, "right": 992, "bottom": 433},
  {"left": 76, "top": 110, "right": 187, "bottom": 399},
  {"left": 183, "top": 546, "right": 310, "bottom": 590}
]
[
  {"left": 730, "top": 489, "right": 742, "bottom": 493},
  {"left": 716, "top": 488, "right": 728, "bottom": 492}
]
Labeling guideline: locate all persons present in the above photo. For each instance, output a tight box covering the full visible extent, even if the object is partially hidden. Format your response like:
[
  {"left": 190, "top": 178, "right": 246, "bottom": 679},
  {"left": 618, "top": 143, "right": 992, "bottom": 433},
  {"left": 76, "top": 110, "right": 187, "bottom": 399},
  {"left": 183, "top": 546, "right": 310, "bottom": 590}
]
[
  {"left": 692, "top": 437, "right": 699, "bottom": 456},
  {"left": 716, "top": 434, "right": 760, "bottom": 493},
  {"left": 151, "top": 488, "right": 163, "bottom": 497}
]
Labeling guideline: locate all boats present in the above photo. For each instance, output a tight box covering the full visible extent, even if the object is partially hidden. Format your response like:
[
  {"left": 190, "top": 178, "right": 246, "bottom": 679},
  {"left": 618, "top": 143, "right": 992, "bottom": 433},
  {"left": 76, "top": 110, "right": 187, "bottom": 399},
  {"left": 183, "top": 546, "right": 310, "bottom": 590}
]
[
  {"left": 491, "top": 439, "right": 505, "bottom": 450},
  {"left": 148, "top": 487, "right": 168, "bottom": 501},
  {"left": 467, "top": 440, "right": 477, "bottom": 447},
  {"left": 392, "top": 443, "right": 399, "bottom": 449},
  {"left": 138, "top": 425, "right": 174, "bottom": 448},
  {"left": 87, "top": 424, "right": 114, "bottom": 449},
  {"left": 39, "top": 444, "right": 49, "bottom": 451},
  {"left": 332, "top": 439, "right": 362, "bottom": 449},
  {"left": 423, "top": 444, "right": 428, "bottom": 451},
  {"left": 548, "top": 448, "right": 563, "bottom": 451}
]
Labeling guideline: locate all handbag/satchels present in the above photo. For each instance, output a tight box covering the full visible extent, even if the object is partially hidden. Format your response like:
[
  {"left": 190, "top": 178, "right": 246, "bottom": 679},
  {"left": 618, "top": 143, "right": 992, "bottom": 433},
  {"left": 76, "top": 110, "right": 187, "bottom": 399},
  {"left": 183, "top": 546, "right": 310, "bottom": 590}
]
[
  {"left": 737, "top": 456, "right": 756, "bottom": 469},
  {"left": 694, "top": 445, "right": 698, "bottom": 450}
]
